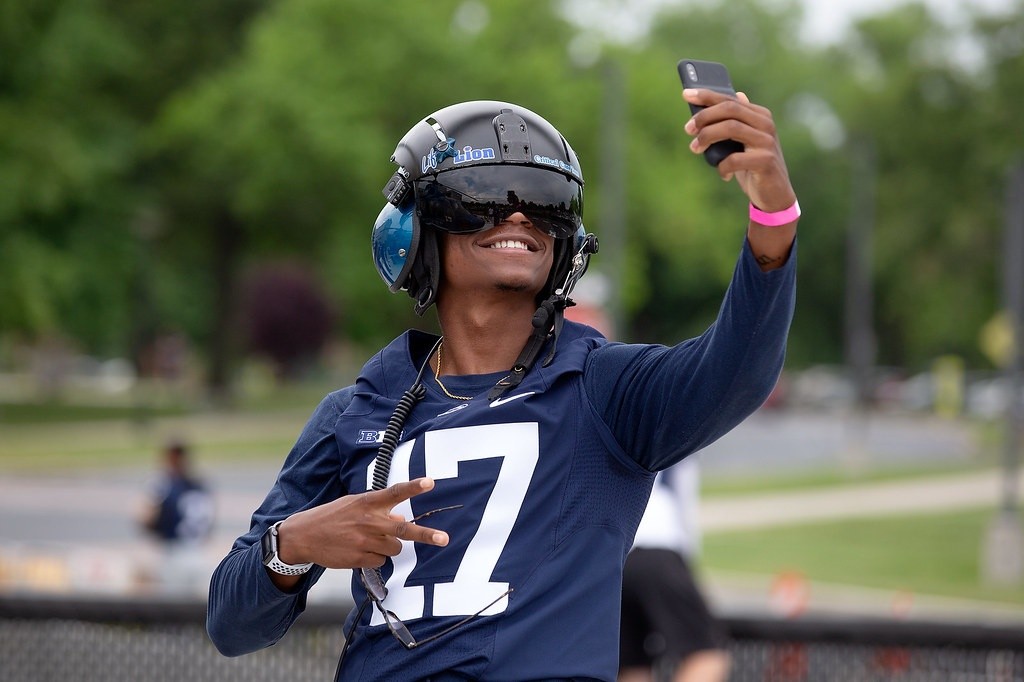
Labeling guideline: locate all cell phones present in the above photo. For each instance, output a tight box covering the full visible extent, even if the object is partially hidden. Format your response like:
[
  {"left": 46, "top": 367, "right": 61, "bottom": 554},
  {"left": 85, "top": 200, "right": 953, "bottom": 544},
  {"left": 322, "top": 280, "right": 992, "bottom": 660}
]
[{"left": 678, "top": 57, "right": 746, "bottom": 167}]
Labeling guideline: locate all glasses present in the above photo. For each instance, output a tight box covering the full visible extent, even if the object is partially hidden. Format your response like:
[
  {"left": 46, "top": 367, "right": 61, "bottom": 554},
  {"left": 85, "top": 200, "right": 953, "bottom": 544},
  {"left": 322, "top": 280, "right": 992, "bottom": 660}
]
[{"left": 358, "top": 503, "right": 514, "bottom": 650}]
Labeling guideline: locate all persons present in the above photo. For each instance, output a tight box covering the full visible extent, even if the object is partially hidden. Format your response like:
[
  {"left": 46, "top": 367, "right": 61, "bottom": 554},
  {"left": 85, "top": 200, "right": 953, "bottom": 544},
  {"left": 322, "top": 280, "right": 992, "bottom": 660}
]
[
  {"left": 620, "top": 455, "right": 730, "bottom": 682},
  {"left": 137, "top": 443, "right": 212, "bottom": 550},
  {"left": 208, "top": 60, "right": 800, "bottom": 682}
]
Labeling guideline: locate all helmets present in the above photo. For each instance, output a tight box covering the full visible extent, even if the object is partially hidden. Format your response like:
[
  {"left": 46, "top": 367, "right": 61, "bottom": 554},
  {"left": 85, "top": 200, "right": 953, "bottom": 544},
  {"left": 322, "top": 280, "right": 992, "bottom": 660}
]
[{"left": 369, "top": 100, "right": 601, "bottom": 317}]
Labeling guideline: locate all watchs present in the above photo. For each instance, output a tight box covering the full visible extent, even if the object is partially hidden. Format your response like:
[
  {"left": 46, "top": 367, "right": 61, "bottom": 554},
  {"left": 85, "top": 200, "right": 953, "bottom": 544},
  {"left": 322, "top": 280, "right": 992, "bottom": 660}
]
[{"left": 261, "top": 520, "right": 314, "bottom": 576}]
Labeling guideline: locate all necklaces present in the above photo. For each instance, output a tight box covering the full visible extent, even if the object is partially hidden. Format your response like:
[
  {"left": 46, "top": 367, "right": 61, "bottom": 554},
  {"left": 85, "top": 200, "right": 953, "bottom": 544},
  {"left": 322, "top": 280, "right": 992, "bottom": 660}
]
[{"left": 435, "top": 343, "right": 513, "bottom": 400}]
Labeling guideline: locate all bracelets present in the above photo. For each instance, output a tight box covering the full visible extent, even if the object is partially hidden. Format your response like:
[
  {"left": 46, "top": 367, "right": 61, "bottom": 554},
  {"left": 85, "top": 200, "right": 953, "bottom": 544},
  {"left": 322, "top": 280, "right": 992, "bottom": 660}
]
[{"left": 750, "top": 199, "right": 802, "bottom": 227}]
